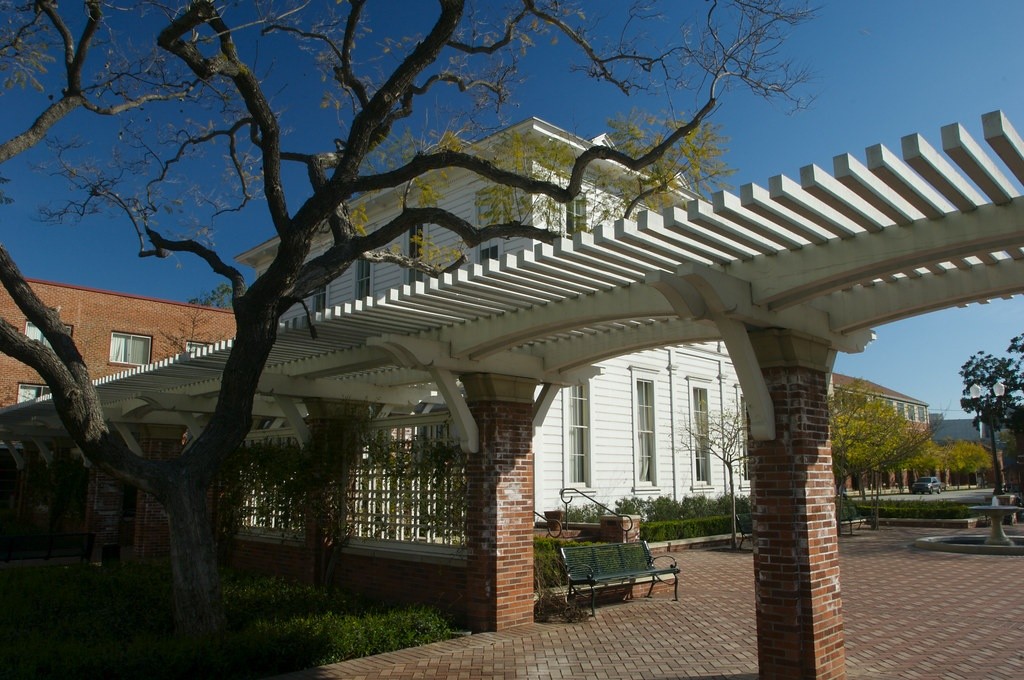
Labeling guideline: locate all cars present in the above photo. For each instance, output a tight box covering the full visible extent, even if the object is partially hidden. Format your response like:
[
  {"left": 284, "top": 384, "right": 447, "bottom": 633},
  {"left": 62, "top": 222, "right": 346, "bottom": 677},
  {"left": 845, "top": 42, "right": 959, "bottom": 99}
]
[{"left": 911, "top": 476, "right": 941, "bottom": 494}]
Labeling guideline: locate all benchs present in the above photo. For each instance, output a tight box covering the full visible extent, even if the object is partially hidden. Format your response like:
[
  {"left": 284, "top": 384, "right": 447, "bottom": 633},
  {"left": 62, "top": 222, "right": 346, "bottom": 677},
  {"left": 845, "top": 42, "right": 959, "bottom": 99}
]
[
  {"left": 557, "top": 540, "right": 680, "bottom": 617},
  {"left": 840, "top": 504, "right": 877, "bottom": 536},
  {"left": 735, "top": 513, "right": 752, "bottom": 551}
]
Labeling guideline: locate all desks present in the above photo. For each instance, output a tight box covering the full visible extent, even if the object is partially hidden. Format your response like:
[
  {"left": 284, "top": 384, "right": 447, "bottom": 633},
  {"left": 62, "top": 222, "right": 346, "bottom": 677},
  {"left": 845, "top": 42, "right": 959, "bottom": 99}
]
[{"left": 966, "top": 505, "right": 1024, "bottom": 546}]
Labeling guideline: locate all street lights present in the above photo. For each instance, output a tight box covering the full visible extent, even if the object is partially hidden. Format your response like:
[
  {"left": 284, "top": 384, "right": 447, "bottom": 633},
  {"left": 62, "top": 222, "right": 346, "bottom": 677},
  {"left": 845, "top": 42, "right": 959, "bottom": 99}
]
[{"left": 970, "top": 380, "right": 1005, "bottom": 495}]
